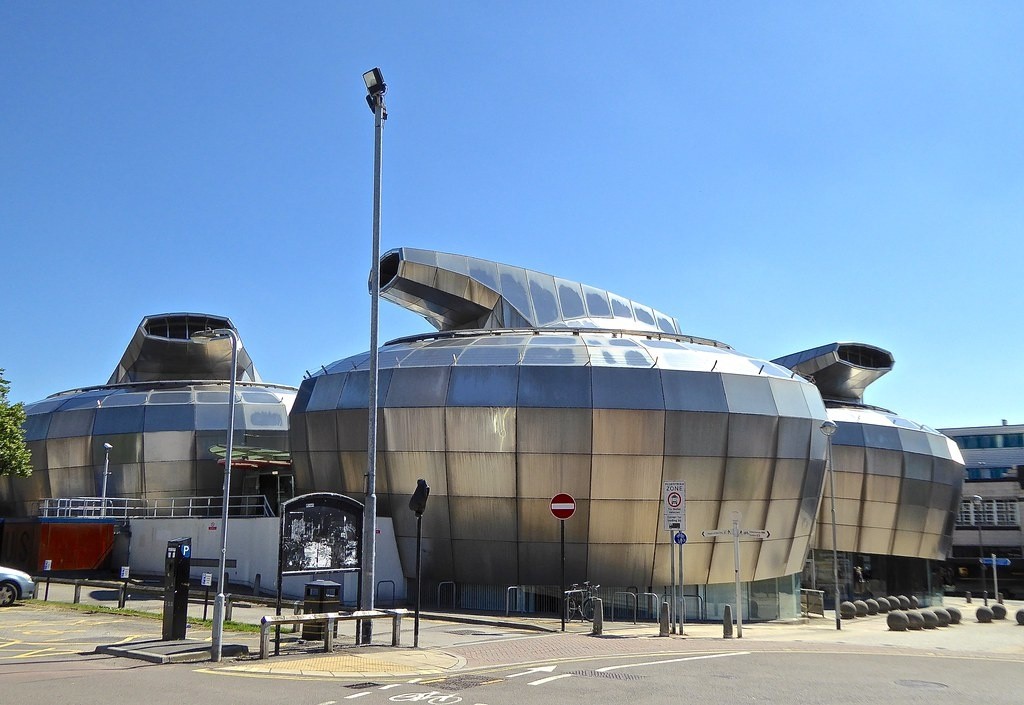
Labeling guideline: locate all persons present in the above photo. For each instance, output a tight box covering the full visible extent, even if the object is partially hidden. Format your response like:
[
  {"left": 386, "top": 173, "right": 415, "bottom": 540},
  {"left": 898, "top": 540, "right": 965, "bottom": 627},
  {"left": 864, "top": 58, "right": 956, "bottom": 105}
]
[{"left": 854, "top": 565, "right": 870, "bottom": 593}]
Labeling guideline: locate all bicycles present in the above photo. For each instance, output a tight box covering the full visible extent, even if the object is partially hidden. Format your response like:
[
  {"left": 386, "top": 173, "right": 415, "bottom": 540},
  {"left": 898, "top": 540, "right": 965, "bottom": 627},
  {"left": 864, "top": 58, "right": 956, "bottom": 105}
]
[{"left": 562, "top": 581, "right": 601, "bottom": 622}]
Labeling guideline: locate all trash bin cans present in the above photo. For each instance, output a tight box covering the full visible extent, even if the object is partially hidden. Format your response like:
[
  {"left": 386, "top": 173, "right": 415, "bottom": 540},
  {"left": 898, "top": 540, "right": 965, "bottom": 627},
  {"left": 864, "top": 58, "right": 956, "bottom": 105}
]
[
  {"left": 302, "top": 580, "right": 342, "bottom": 641},
  {"left": 800, "top": 589, "right": 825, "bottom": 619}
]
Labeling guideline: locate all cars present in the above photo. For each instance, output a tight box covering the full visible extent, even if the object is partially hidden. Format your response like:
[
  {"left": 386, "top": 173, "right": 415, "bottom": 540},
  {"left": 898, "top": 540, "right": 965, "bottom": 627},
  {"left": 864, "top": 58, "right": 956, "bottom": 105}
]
[{"left": 0, "top": 564, "right": 36, "bottom": 607}]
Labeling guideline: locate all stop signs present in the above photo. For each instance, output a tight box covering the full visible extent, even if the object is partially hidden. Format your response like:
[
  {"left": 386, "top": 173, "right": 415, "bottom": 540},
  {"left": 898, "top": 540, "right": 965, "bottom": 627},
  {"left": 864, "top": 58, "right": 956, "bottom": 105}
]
[{"left": 549, "top": 493, "right": 577, "bottom": 520}]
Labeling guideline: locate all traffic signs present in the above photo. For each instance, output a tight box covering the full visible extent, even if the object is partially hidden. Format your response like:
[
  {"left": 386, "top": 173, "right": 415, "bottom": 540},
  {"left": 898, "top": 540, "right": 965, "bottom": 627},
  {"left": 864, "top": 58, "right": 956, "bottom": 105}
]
[{"left": 978, "top": 557, "right": 1011, "bottom": 566}]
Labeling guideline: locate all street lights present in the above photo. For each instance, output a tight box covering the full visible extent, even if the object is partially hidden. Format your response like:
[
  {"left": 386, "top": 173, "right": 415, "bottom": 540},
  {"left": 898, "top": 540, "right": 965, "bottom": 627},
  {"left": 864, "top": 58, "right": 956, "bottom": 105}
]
[
  {"left": 100, "top": 442, "right": 114, "bottom": 517},
  {"left": 361, "top": 67, "right": 388, "bottom": 648},
  {"left": 971, "top": 493, "right": 989, "bottom": 606},
  {"left": 190, "top": 327, "right": 237, "bottom": 664}
]
